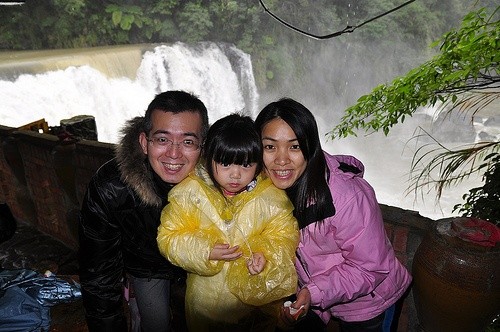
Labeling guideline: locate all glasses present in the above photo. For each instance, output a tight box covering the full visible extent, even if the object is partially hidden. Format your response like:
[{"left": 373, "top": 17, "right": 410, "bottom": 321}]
[{"left": 147, "top": 137, "right": 203, "bottom": 151}]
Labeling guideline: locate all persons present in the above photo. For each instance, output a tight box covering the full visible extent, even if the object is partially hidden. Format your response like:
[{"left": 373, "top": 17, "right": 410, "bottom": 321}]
[
  {"left": 254, "top": 98, "right": 412, "bottom": 332},
  {"left": 156, "top": 114, "right": 300, "bottom": 332},
  {"left": 79, "top": 91, "right": 209, "bottom": 332}
]
